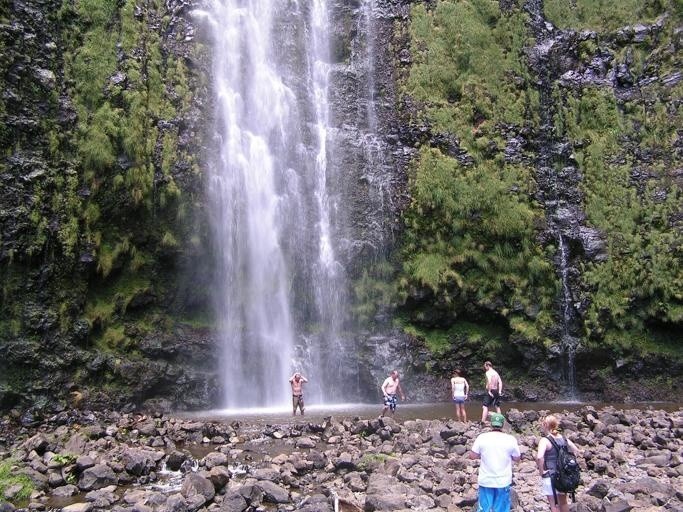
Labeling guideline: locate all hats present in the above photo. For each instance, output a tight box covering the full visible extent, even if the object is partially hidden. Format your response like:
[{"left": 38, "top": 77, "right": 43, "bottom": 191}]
[{"left": 491, "top": 414, "right": 505, "bottom": 427}]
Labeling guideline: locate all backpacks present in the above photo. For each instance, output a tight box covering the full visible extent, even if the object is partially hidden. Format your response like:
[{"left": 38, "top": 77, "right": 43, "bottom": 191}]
[{"left": 548, "top": 434, "right": 579, "bottom": 493}]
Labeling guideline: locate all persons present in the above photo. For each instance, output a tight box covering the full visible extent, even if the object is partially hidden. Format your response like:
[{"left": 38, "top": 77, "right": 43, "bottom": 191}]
[
  {"left": 469, "top": 413, "right": 521, "bottom": 512},
  {"left": 536, "top": 414, "right": 569, "bottom": 512},
  {"left": 451, "top": 369, "right": 469, "bottom": 423},
  {"left": 378, "top": 370, "right": 405, "bottom": 420},
  {"left": 289, "top": 373, "right": 308, "bottom": 418},
  {"left": 477, "top": 361, "right": 503, "bottom": 426}
]
